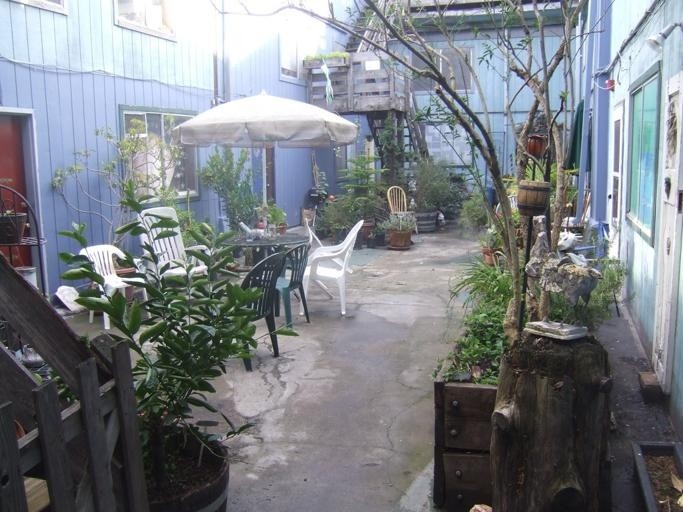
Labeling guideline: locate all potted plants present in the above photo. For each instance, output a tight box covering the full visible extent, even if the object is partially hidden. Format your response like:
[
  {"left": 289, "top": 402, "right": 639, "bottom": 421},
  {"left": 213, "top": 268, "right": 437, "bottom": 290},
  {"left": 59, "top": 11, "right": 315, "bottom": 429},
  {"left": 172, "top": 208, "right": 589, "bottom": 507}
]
[
  {"left": 56, "top": 180, "right": 297, "bottom": 507},
  {"left": 413, "top": 164, "right": 449, "bottom": 234},
  {"left": 366, "top": 214, "right": 415, "bottom": 250}
]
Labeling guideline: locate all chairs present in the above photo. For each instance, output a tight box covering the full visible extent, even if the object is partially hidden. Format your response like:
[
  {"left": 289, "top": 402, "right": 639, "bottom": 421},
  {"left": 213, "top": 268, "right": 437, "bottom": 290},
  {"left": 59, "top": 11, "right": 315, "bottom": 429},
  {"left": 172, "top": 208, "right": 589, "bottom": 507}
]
[
  {"left": 387, "top": 186, "right": 418, "bottom": 236},
  {"left": 75, "top": 206, "right": 363, "bottom": 371}
]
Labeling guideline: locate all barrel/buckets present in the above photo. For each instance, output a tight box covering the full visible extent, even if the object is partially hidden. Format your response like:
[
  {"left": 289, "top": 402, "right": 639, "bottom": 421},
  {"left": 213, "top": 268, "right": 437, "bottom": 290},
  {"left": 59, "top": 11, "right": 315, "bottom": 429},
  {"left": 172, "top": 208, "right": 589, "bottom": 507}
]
[
  {"left": 412, "top": 209, "right": 438, "bottom": 233},
  {"left": 147, "top": 424, "right": 231, "bottom": 512},
  {"left": 517, "top": 180, "right": 550, "bottom": 216}
]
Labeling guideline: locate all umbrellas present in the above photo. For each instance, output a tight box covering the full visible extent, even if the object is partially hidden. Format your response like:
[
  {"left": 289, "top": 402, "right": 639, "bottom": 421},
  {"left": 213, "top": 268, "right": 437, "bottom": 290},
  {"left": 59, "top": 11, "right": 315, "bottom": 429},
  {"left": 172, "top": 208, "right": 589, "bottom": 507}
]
[{"left": 172, "top": 89, "right": 358, "bottom": 228}]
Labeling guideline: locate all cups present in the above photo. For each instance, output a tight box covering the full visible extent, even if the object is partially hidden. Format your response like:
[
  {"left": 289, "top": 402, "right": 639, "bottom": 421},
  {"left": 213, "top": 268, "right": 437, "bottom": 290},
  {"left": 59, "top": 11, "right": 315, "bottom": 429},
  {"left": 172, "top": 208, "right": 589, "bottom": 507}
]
[
  {"left": 234, "top": 235, "right": 241, "bottom": 244},
  {"left": 264, "top": 223, "right": 276, "bottom": 240}
]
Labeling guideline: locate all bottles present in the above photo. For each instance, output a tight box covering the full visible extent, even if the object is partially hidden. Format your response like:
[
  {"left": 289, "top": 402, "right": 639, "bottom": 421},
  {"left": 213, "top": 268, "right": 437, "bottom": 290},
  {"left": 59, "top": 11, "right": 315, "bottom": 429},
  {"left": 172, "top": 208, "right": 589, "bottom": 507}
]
[{"left": 257, "top": 220, "right": 264, "bottom": 238}]
